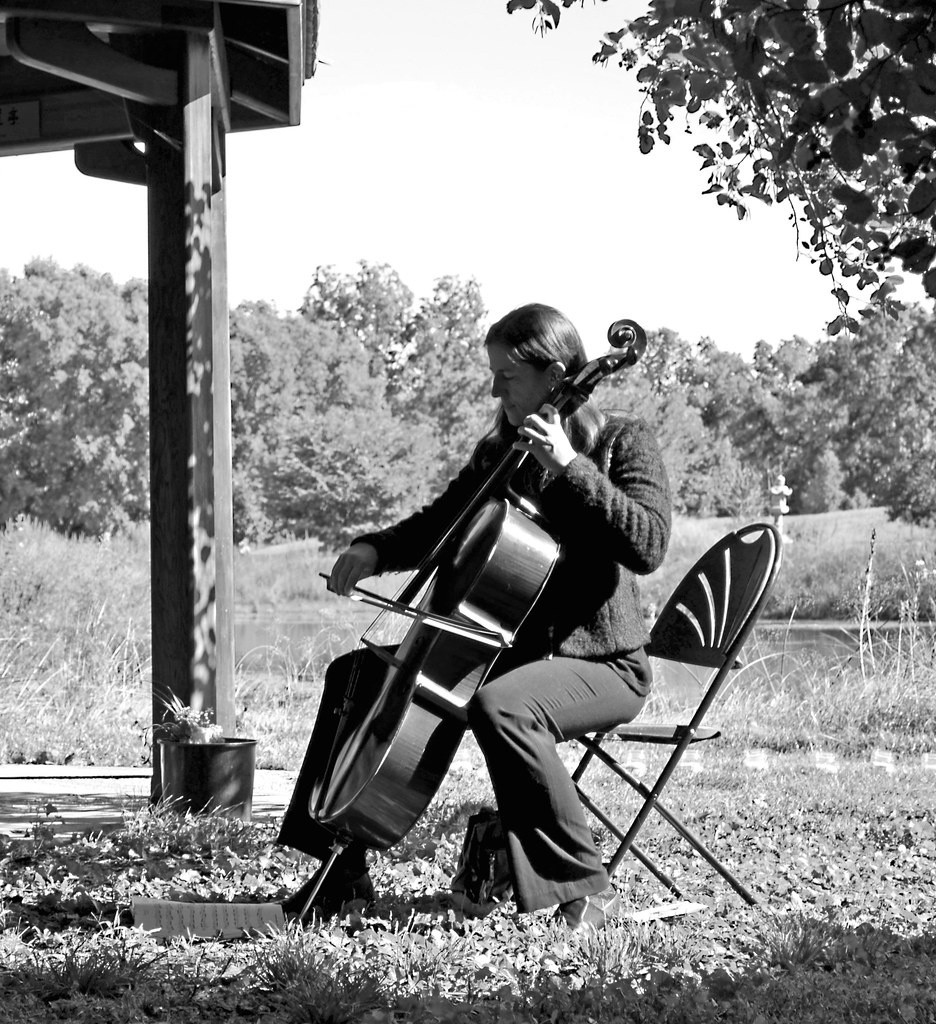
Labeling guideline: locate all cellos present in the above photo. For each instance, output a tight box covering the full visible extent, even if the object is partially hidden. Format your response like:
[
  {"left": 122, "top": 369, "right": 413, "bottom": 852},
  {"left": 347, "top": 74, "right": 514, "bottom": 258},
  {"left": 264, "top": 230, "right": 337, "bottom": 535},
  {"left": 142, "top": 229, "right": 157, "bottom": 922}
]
[{"left": 258, "top": 313, "right": 651, "bottom": 986}]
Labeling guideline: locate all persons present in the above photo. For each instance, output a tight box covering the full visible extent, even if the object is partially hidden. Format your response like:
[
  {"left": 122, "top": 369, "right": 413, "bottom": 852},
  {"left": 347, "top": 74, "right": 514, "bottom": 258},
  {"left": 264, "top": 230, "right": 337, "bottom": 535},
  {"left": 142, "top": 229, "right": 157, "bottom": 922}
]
[{"left": 278, "top": 302, "right": 671, "bottom": 931}]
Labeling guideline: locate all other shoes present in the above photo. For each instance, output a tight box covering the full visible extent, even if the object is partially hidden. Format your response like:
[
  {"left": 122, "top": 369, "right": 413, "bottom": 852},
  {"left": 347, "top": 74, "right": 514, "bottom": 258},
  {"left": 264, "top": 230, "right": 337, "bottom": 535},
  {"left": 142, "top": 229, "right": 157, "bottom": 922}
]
[
  {"left": 280, "top": 869, "right": 377, "bottom": 915},
  {"left": 546, "top": 883, "right": 622, "bottom": 931}
]
